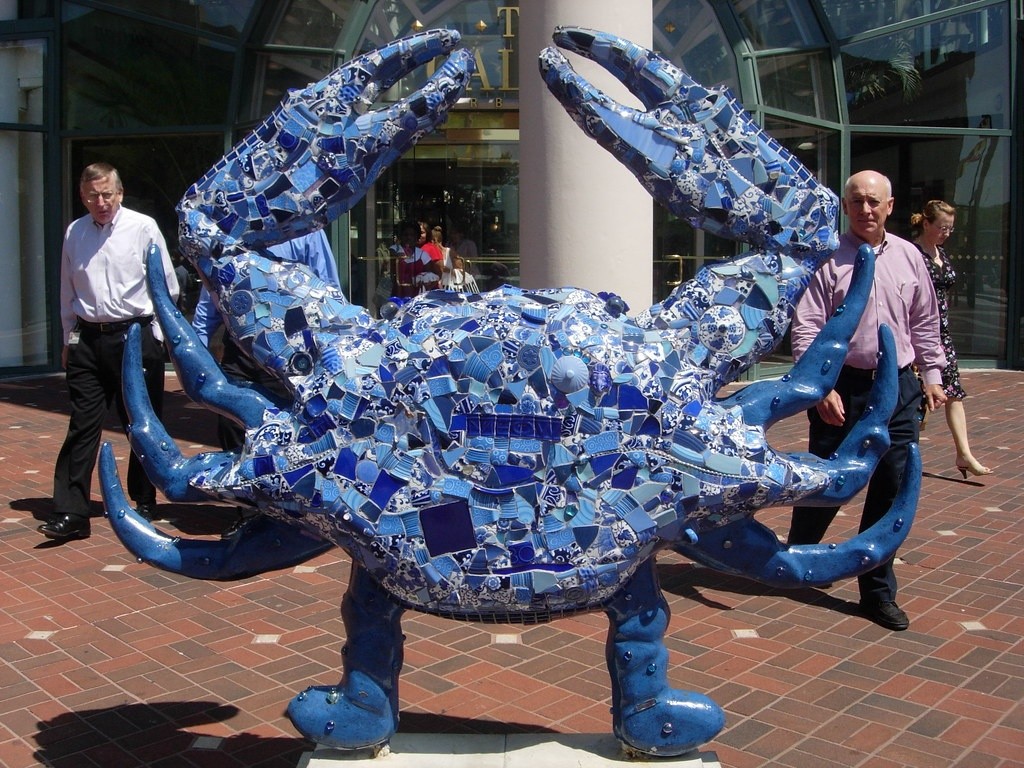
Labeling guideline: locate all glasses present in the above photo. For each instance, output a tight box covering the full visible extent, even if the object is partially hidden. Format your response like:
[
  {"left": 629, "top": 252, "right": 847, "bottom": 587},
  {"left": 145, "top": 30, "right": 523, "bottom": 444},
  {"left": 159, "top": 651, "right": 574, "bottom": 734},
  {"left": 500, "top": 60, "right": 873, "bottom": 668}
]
[
  {"left": 939, "top": 225, "right": 956, "bottom": 232},
  {"left": 81, "top": 188, "right": 121, "bottom": 202}
]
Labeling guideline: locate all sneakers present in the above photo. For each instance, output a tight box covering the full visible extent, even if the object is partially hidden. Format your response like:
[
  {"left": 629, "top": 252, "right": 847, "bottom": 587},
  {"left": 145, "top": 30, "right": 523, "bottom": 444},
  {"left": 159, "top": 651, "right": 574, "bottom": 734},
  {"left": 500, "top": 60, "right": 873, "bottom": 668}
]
[{"left": 859, "top": 601, "right": 909, "bottom": 630}]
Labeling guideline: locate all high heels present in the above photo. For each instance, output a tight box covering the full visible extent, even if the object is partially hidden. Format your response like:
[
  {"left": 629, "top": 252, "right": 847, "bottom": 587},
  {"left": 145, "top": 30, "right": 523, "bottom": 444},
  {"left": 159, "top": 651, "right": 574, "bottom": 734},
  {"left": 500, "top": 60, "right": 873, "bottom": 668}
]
[{"left": 957, "top": 461, "right": 993, "bottom": 479}]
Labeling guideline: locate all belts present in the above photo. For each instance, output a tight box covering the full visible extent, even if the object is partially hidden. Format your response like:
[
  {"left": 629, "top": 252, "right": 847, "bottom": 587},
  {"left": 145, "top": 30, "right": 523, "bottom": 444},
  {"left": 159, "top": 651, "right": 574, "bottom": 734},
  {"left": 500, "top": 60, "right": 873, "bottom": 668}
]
[
  {"left": 77, "top": 315, "right": 153, "bottom": 333},
  {"left": 841, "top": 363, "right": 911, "bottom": 381}
]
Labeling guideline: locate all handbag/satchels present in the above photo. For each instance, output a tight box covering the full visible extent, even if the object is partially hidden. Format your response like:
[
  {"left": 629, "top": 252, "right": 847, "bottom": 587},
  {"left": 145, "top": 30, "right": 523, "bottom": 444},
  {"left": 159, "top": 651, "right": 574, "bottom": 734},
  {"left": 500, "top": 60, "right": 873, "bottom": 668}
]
[
  {"left": 372, "top": 276, "right": 395, "bottom": 309},
  {"left": 443, "top": 268, "right": 482, "bottom": 295}
]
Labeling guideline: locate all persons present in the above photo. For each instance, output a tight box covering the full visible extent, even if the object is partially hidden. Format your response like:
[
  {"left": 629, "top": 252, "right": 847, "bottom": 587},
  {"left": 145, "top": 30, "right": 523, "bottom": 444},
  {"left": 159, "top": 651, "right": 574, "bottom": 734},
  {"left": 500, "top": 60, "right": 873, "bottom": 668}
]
[
  {"left": 190, "top": 228, "right": 343, "bottom": 539},
  {"left": 906, "top": 200, "right": 992, "bottom": 478},
  {"left": 390, "top": 219, "right": 512, "bottom": 294},
  {"left": 37, "top": 163, "right": 180, "bottom": 538},
  {"left": 785, "top": 170, "right": 949, "bottom": 629}
]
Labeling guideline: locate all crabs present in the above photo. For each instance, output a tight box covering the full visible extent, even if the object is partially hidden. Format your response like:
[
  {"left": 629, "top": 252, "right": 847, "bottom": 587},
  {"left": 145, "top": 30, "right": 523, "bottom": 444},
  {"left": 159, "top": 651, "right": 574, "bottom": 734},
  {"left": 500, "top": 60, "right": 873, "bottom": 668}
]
[{"left": 98, "top": 27, "right": 924, "bottom": 758}]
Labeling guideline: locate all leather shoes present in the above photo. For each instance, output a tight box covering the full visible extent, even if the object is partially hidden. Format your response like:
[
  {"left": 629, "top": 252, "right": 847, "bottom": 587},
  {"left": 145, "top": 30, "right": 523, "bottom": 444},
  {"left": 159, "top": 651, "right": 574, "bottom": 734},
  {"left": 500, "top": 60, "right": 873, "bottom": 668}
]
[
  {"left": 37, "top": 513, "right": 91, "bottom": 537},
  {"left": 134, "top": 502, "right": 158, "bottom": 523},
  {"left": 220, "top": 515, "right": 259, "bottom": 540}
]
